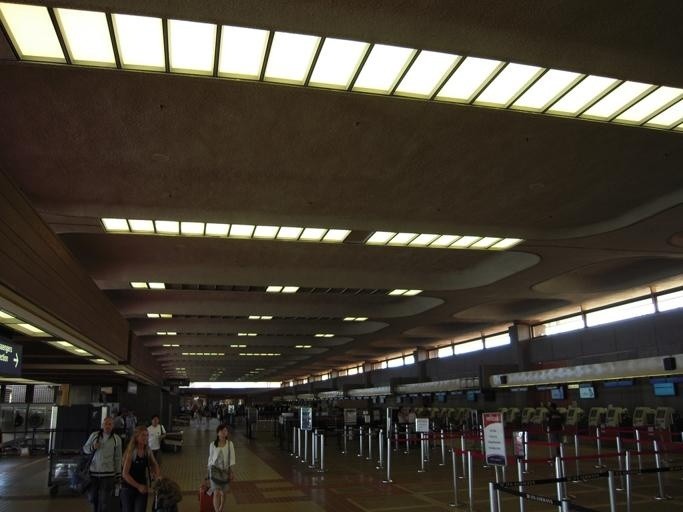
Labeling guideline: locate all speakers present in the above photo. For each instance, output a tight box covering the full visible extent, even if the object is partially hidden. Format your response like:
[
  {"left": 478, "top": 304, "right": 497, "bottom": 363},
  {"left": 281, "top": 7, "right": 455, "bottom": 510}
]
[
  {"left": 499, "top": 375, "right": 506, "bottom": 384},
  {"left": 663, "top": 358, "right": 676, "bottom": 370}
]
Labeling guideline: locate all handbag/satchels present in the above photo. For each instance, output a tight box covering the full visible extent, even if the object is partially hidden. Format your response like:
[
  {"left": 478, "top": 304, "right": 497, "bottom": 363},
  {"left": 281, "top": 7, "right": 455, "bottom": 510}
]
[
  {"left": 69, "top": 471, "right": 90, "bottom": 496},
  {"left": 160, "top": 424, "right": 167, "bottom": 453},
  {"left": 210, "top": 465, "right": 230, "bottom": 484}
]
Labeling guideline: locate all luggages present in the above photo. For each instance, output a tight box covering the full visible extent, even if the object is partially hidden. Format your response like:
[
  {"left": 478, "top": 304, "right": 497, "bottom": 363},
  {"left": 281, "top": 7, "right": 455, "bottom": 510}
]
[
  {"left": 198, "top": 484, "right": 223, "bottom": 511},
  {"left": 152, "top": 488, "right": 177, "bottom": 512}
]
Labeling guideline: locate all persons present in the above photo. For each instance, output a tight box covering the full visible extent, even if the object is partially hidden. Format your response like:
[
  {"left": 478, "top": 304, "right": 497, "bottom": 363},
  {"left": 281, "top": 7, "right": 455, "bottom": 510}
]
[
  {"left": 397, "top": 406, "right": 417, "bottom": 447},
  {"left": 207, "top": 424, "right": 235, "bottom": 512},
  {"left": 544, "top": 403, "right": 566, "bottom": 462},
  {"left": 83, "top": 407, "right": 166, "bottom": 512}
]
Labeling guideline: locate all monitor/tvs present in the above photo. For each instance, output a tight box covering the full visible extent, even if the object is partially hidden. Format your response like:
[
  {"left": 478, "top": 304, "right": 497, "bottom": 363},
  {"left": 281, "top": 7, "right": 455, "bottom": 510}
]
[
  {"left": 372, "top": 398, "right": 377, "bottom": 403},
  {"left": 579, "top": 383, "right": 597, "bottom": 400},
  {"left": 379, "top": 397, "right": 384, "bottom": 402},
  {"left": 438, "top": 395, "right": 446, "bottom": 402},
  {"left": 653, "top": 376, "right": 676, "bottom": 398},
  {"left": 466, "top": 392, "right": 476, "bottom": 401},
  {"left": 551, "top": 385, "right": 565, "bottom": 401}
]
